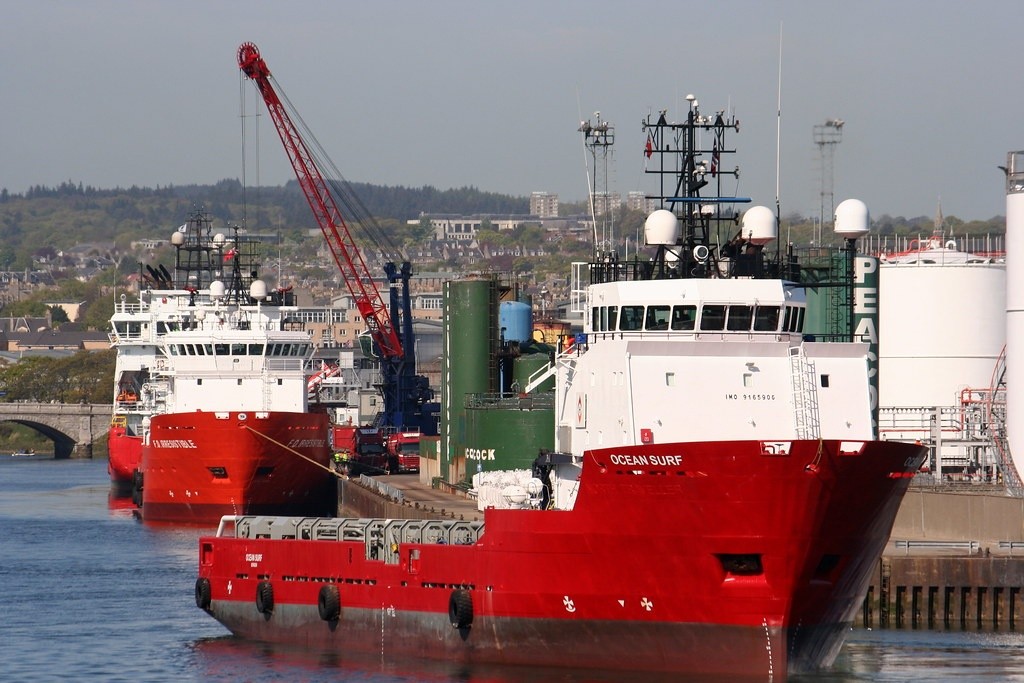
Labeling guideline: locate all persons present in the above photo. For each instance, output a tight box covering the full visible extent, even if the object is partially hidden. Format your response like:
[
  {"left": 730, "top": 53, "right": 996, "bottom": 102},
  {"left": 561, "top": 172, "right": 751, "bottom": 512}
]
[
  {"left": 342, "top": 451, "right": 348, "bottom": 461},
  {"left": 334, "top": 452, "right": 340, "bottom": 462}
]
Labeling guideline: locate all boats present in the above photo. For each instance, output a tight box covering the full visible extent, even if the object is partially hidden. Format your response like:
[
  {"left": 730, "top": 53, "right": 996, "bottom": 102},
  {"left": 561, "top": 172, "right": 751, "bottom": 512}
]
[
  {"left": 10, "top": 451, "right": 35, "bottom": 457},
  {"left": 194, "top": 93, "right": 930, "bottom": 683},
  {"left": 108, "top": 213, "right": 332, "bottom": 527}
]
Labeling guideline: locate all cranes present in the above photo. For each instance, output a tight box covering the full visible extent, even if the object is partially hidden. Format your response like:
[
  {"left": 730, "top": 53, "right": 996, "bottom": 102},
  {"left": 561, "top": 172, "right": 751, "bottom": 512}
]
[{"left": 240, "top": 42, "right": 441, "bottom": 441}]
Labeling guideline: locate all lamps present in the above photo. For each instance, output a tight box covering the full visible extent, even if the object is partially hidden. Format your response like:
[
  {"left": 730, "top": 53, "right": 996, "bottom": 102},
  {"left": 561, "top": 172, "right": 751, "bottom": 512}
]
[{"left": 691, "top": 244, "right": 710, "bottom": 264}]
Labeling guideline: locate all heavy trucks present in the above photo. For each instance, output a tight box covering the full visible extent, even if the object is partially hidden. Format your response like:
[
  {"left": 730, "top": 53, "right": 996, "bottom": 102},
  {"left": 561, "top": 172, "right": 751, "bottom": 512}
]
[{"left": 331, "top": 426, "right": 424, "bottom": 475}]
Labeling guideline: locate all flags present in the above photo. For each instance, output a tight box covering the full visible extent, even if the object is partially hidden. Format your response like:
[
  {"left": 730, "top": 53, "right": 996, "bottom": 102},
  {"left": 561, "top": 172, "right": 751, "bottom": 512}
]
[
  {"left": 646, "top": 136, "right": 652, "bottom": 159},
  {"left": 711, "top": 138, "right": 719, "bottom": 178},
  {"left": 225, "top": 247, "right": 236, "bottom": 262}
]
